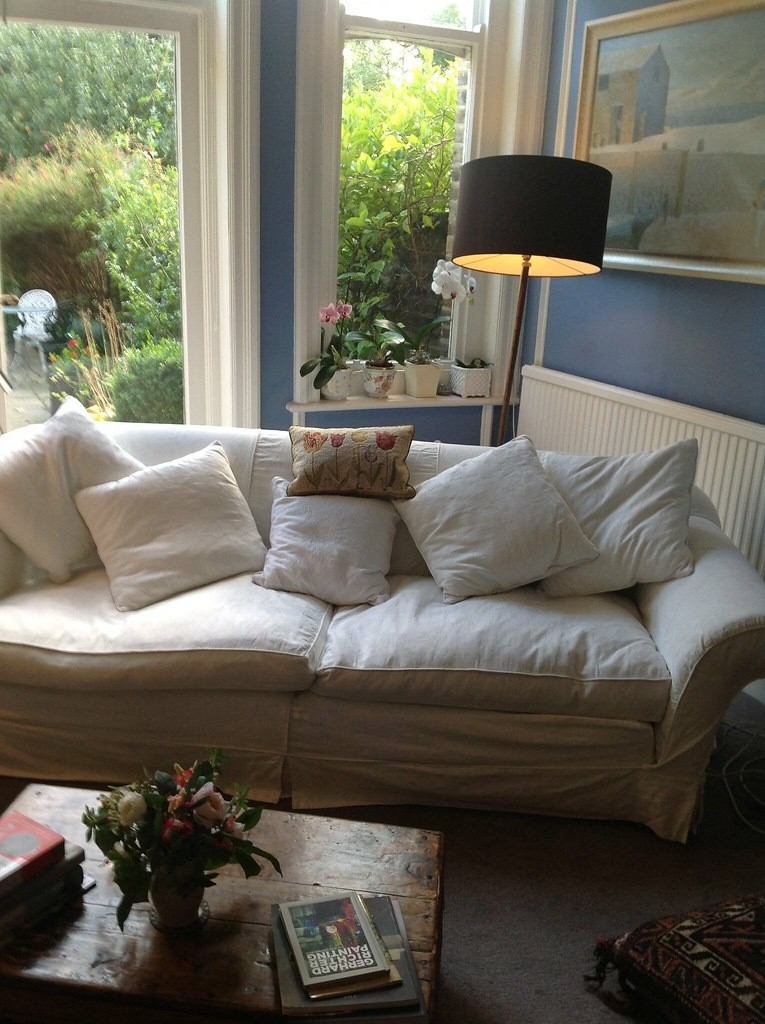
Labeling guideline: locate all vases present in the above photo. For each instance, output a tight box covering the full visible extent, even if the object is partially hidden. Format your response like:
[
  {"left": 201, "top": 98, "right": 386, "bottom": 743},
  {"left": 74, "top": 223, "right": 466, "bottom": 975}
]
[
  {"left": 148, "top": 874, "right": 211, "bottom": 933},
  {"left": 319, "top": 367, "right": 353, "bottom": 401},
  {"left": 403, "top": 361, "right": 444, "bottom": 398},
  {"left": 45, "top": 372, "right": 90, "bottom": 418}
]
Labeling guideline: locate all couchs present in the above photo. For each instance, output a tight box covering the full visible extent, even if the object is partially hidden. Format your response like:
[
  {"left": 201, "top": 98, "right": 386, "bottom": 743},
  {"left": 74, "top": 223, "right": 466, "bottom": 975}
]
[{"left": 0, "top": 417, "right": 765, "bottom": 845}]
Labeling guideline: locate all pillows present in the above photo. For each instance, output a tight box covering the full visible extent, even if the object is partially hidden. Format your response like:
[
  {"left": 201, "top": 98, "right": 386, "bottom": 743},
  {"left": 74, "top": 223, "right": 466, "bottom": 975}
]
[
  {"left": 587, "top": 884, "right": 765, "bottom": 1024},
  {"left": 0, "top": 395, "right": 148, "bottom": 588},
  {"left": 250, "top": 476, "right": 402, "bottom": 606},
  {"left": 395, "top": 435, "right": 604, "bottom": 609},
  {"left": 543, "top": 437, "right": 700, "bottom": 599},
  {"left": 284, "top": 426, "right": 417, "bottom": 502},
  {"left": 70, "top": 438, "right": 268, "bottom": 615}
]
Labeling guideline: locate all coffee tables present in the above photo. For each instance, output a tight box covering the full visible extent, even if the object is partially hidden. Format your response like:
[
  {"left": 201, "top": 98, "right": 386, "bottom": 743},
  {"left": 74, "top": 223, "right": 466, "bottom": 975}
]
[{"left": 0, "top": 783, "right": 446, "bottom": 1024}]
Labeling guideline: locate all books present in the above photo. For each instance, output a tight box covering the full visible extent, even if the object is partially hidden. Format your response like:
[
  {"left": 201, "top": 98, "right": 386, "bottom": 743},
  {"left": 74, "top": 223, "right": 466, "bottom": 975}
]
[
  {"left": 0, "top": 811, "right": 96, "bottom": 951},
  {"left": 271, "top": 893, "right": 427, "bottom": 1024}
]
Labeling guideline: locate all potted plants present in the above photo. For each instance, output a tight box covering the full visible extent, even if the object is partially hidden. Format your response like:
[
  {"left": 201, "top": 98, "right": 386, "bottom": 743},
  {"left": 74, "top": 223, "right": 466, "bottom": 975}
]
[
  {"left": 450, "top": 357, "right": 492, "bottom": 399},
  {"left": 344, "top": 311, "right": 406, "bottom": 399}
]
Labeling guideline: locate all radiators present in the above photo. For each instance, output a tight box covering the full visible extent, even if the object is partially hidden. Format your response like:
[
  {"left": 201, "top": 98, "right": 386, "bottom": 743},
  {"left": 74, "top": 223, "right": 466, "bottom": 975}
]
[{"left": 516, "top": 364, "right": 765, "bottom": 709}]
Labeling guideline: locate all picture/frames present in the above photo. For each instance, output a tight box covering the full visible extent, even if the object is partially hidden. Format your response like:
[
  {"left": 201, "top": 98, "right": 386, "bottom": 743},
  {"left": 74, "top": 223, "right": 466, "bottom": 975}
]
[{"left": 570, "top": 0, "right": 765, "bottom": 287}]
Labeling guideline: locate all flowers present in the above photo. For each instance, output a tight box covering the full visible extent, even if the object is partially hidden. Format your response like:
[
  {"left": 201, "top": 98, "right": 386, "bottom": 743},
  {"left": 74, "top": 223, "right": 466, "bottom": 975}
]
[
  {"left": 298, "top": 299, "right": 353, "bottom": 388},
  {"left": 48, "top": 330, "right": 102, "bottom": 372},
  {"left": 430, "top": 259, "right": 477, "bottom": 306},
  {"left": 82, "top": 755, "right": 284, "bottom": 936}
]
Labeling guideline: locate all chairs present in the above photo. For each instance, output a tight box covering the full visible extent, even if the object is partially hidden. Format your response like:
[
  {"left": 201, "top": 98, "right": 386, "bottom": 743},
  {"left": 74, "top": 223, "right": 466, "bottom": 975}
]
[
  {"left": 35, "top": 298, "right": 83, "bottom": 364},
  {"left": 8, "top": 288, "right": 59, "bottom": 375}
]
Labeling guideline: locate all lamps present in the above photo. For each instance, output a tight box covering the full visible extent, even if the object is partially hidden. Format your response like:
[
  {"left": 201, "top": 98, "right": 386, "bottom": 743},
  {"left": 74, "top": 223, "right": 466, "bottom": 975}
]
[{"left": 452, "top": 154, "right": 613, "bottom": 447}]
